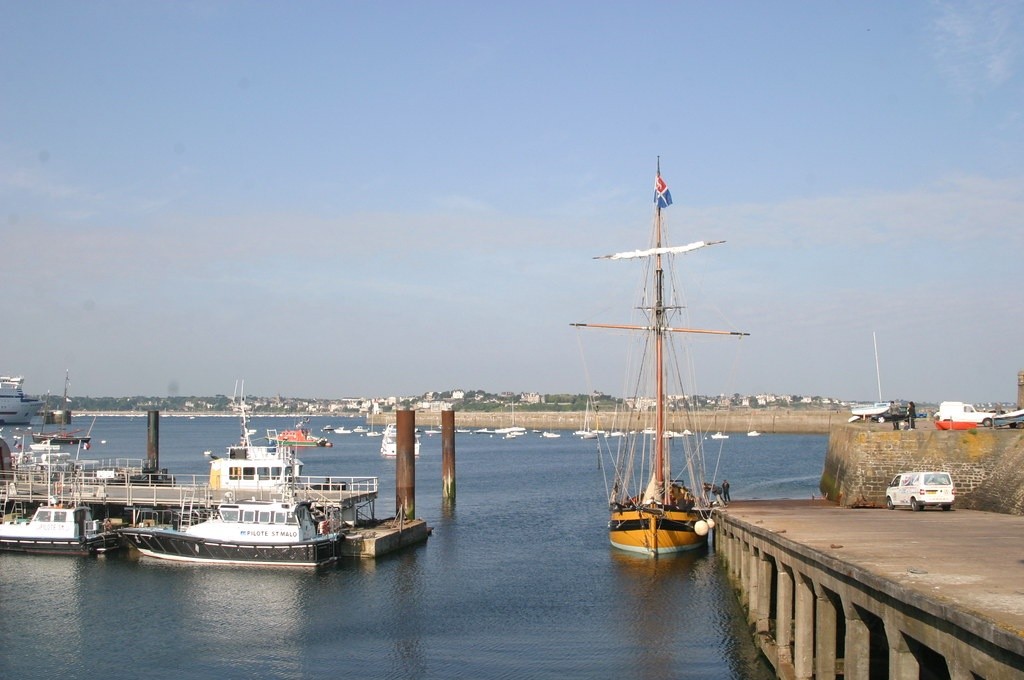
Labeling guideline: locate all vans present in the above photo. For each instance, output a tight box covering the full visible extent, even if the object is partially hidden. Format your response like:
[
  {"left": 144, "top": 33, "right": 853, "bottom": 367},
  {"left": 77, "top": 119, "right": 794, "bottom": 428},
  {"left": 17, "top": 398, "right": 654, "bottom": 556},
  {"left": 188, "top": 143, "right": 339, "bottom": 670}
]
[{"left": 886, "top": 472, "right": 955, "bottom": 511}]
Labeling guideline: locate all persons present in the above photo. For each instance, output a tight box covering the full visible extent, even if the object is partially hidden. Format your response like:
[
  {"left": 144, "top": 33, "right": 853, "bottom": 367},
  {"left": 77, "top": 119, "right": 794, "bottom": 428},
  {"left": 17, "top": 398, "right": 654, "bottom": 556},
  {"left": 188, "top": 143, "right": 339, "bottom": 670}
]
[
  {"left": 712, "top": 484, "right": 725, "bottom": 507},
  {"left": 907, "top": 402, "right": 916, "bottom": 428},
  {"left": 890, "top": 401, "right": 900, "bottom": 430},
  {"left": 723, "top": 480, "right": 730, "bottom": 502}
]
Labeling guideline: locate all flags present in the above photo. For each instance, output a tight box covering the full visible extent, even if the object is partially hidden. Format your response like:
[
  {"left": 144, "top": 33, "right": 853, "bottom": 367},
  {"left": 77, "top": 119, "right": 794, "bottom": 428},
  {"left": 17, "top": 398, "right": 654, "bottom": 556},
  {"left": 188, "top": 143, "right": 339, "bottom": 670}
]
[
  {"left": 654, "top": 174, "right": 673, "bottom": 209},
  {"left": 14, "top": 441, "right": 22, "bottom": 450},
  {"left": 80, "top": 441, "right": 90, "bottom": 451}
]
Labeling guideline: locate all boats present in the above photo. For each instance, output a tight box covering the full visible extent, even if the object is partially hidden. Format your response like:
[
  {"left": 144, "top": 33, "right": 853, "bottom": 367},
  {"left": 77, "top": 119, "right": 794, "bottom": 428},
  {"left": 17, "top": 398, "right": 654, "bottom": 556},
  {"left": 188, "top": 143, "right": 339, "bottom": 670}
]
[
  {"left": 0, "top": 494, "right": 116, "bottom": 555},
  {"left": 266, "top": 422, "right": 333, "bottom": 449},
  {"left": 473, "top": 428, "right": 496, "bottom": 434},
  {"left": 379, "top": 422, "right": 419, "bottom": 457},
  {"left": 455, "top": 429, "right": 470, "bottom": 433},
  {"left": 425, "top": 430, "right": 442, "bottom": 435},
  {"left": 114, "top": 495, "right": 347, "bottom": 568},
  {"left": 711, "top": 432, "right": 729, "bottom": 439},
  {"left": 574, "top": 426, "right": 692, "bottom": 440},
  {"left": 495, "top": 425, "right": 561, "bottom": 440},
  {"left": 29, "top": 440, "right": 60, "bottom": 450},
  {"left": 747, "top": 431, "right": 761, "bottom": 437},
  {"left": 0, "top": 373, "right": 43, "bottom": 423},
  {"left": 324, "top": 424, "right": 382, "bottom": 437},
  {"left": 936, "top": 420, "right": 977, "bottom": 430}
]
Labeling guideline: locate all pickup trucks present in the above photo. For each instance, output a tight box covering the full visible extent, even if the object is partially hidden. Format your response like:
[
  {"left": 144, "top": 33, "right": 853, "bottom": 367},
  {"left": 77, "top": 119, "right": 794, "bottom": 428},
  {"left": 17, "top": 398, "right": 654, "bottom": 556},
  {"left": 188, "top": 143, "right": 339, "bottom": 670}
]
[{"left": 940, "top": 401, "right": 996, "bottom": 426}]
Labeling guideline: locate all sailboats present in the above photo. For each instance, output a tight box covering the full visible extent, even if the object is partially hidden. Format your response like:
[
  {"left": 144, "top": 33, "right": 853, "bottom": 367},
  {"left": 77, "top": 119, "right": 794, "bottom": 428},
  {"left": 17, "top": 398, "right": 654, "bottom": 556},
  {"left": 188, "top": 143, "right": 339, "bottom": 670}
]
[
  {"left": 570, "top": 154, "right": 752, "bottom": 557},
  {"left": 31, "top": 368, "right": 96, "bottom": 444},
  {"left": 207, "top": 380, "right": 303, "bottom": 488}
]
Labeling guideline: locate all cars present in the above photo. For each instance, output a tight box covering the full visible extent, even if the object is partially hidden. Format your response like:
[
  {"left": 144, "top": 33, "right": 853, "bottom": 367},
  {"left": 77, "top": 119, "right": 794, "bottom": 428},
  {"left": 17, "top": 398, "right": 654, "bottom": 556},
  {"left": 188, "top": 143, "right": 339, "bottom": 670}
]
[{"left": 872, "top": 405, "right": 911, "bottom": 422}]
